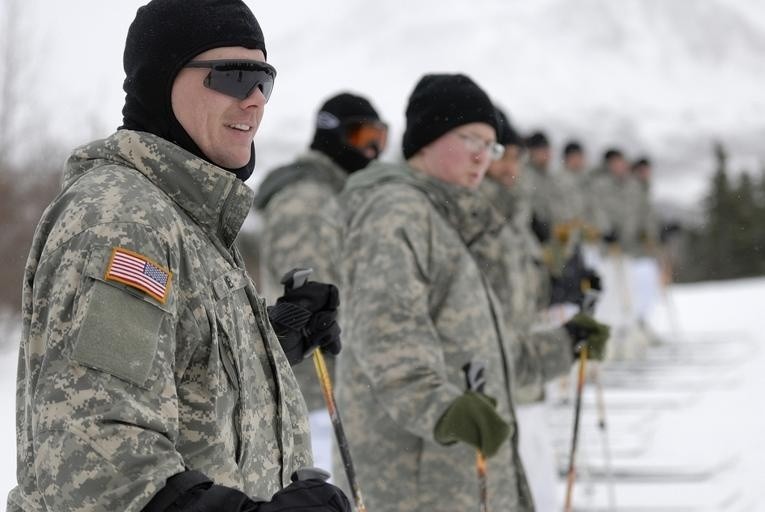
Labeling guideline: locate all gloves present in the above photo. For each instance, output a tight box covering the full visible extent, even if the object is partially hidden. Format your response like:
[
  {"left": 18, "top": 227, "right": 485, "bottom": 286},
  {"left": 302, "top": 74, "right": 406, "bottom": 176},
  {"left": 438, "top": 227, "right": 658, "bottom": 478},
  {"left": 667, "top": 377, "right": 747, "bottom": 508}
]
[
  {"left": 552, "top": 270, "right": 600, "bottom": 303},
  {"left": 144, "top": 466, "right": 351, "bottom": 512},
  {"left": 268, "top": 281, "right": 340, "bottom": 366},
  {"left": 566, "top": 314, "right": 608, "bottom": 359},
  {"left": 435, "top": 390, "right": 510, "bottom": 457}
]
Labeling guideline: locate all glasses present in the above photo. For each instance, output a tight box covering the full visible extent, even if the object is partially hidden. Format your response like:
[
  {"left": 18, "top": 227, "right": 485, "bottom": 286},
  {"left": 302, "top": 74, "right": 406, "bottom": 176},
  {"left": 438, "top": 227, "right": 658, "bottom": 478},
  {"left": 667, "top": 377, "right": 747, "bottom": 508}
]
[
  {"left": 454, "top": 128, "right": 505, "bottom": 159},
  {"left": 316, "top": 112, "right": 387, "bottom": 159},
  {"left": 186, "top": 58, "right": 276, "bottom": 104}
]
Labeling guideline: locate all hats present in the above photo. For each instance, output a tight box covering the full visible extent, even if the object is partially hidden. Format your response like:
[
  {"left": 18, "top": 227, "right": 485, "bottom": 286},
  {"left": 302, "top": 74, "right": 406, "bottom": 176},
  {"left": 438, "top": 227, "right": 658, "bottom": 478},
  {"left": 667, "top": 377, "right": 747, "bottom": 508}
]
[
  {"left": 309, "top": 94, "right": 379, "bottom": 173},
  {"left": 402, "top": 74, "right": 499, "bottom": 159},
  {"left": 565, "top": 145, "right": 647, "bottom": 168},
  {"left": 116, "top": 1, "right": 266, "bottom": 181},
  {"left": 496, "top": 107, "right": 547, "bottom": 147}
]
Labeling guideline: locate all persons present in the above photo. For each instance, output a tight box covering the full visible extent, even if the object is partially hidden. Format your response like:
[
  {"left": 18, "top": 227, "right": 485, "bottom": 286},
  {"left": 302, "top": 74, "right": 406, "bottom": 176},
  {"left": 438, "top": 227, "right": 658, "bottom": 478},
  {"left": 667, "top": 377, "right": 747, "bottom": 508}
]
[
  {"left": 253, "top": 91, "right": 389, "bottom": 472},
  {"left": 319, "top": 73, "right": 610, "bottom": 511},
  {"left": 479, "top": 127, "right": 672, "bottom": 402},
  {"left": 6, "top": 0, "right": 351, "bottom": 512}
]
